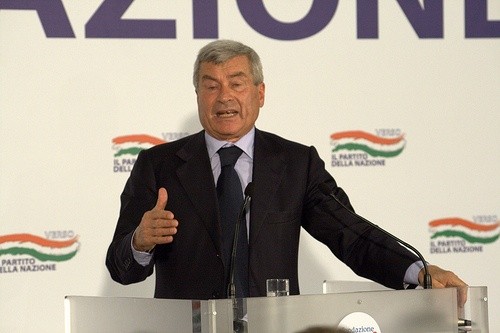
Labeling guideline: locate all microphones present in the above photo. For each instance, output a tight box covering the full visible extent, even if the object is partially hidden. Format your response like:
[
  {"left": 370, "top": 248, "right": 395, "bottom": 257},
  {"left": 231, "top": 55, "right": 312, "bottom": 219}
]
[
  {"left": 227, "top": 182, "right": 256, "bottom": 299},
  {"left": 317, "top": 182, "right": 431, "bottom": 289}
]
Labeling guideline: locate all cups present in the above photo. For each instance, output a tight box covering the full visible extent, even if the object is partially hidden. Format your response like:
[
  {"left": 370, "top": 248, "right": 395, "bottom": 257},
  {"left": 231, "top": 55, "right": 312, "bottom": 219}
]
[{"left": 266, "top": 279, "right": 290, "bottom": 297}]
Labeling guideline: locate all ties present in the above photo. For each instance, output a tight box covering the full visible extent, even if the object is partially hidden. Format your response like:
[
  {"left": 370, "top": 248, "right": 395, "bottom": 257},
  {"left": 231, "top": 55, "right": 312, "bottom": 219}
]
[{"left": 215, "top": 146, "right": 250, "bottom": 297}]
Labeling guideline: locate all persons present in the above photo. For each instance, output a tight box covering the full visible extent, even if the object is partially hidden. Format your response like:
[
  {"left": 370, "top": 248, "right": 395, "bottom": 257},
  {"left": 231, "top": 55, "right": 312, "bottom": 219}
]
[{"left": 105, "top": 38, "right": 472, "bottom": 310}]
